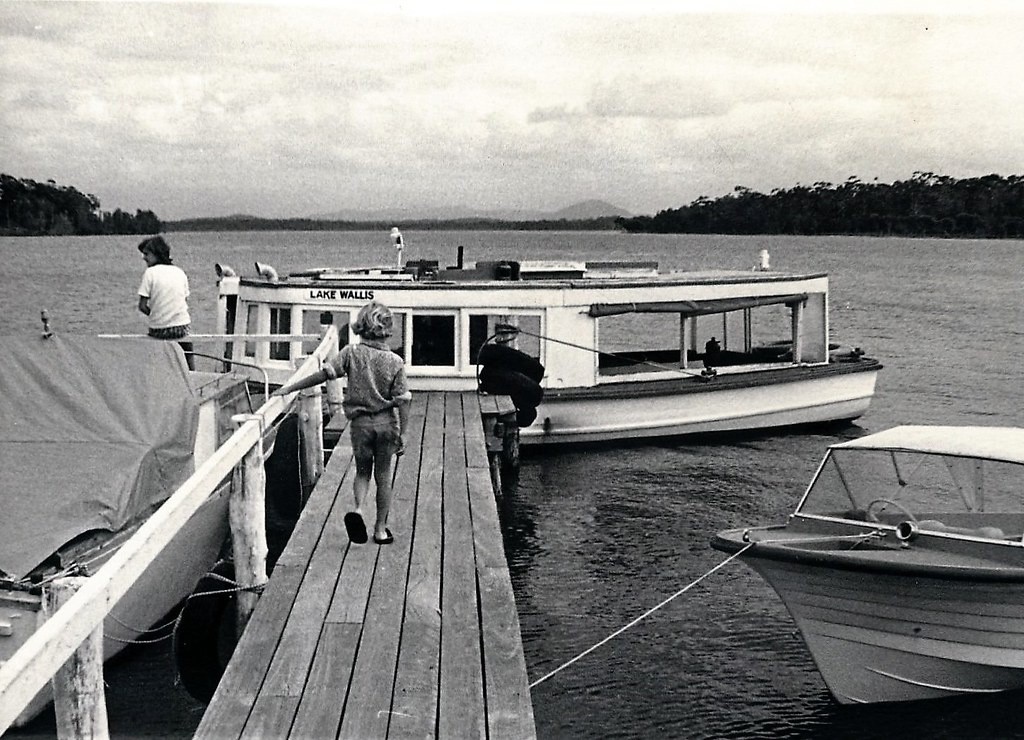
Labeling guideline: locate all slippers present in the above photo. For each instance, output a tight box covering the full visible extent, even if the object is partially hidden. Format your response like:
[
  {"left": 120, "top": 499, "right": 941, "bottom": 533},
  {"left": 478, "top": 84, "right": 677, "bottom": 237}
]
[
  {"left": 373, "top": 528, "right": 393, "bottom": 545},
  {"left": 344, "top": 512, "right": 368, "bottom": 544}
]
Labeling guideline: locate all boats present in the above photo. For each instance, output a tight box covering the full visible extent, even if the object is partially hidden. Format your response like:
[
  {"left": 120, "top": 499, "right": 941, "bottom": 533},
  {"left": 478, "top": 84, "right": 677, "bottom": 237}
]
[
  {"left": 709, "top": 424, "right": 1024, "bottom": 716},
  {"left": 0, "top": 311, "right": 302, "bottom": 737},
  {"left": 213, "top": 227, "right": 884, "bottom": 459}
]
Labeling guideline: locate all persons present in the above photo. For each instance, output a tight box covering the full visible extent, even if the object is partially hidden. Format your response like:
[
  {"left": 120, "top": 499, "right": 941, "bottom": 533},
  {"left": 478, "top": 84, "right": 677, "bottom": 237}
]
[
  {"left": 138, "top": 235, "right": 193, "bottom": 371},
  {"left": 272, "top": 302, "right": 412, "bottom": 544}
]
